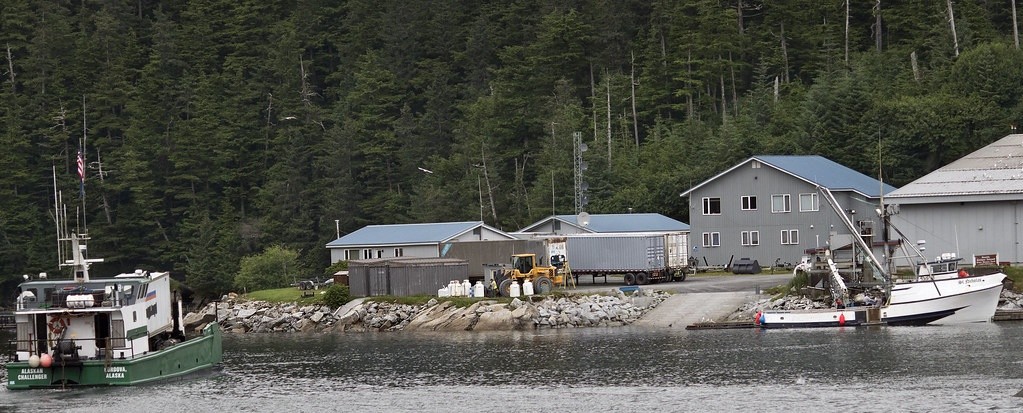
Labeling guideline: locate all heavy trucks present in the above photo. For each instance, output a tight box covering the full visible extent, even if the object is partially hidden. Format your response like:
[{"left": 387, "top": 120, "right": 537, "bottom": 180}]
[{"left": 547, "top": 232, "right": 686, "bottom": 286}]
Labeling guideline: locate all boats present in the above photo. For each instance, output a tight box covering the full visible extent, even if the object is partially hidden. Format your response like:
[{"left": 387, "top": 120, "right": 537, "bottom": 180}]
[
  {"left": 3, "top": 163, "right": 224, "bottom": 391},
  {"left": 758, "top": 184, "right": 1008, "bottom": 330}
]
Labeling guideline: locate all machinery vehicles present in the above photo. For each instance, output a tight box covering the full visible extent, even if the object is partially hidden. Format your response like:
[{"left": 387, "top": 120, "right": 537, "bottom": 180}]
[{"left": 481, "top": 252, "right": 568, "bottom": 299}]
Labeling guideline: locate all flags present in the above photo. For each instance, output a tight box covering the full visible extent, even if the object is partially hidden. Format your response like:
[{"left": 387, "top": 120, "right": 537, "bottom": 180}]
[{"left": 77, "top": 147, "right": 83, "bottom": 178}]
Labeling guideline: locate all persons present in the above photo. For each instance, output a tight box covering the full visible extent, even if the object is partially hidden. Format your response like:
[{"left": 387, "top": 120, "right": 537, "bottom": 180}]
[
  {"left": 490, "top": 279, "right": 497, "bottom": 298},
  {"left": 816, "top": 255, "right": 821, "bottom": 270},
  {"left": 862, "top": 293, "right": 887, "bottom": 306},
  {"left": 959, "top": 269, "right": 969, "bottom": 278}
]
